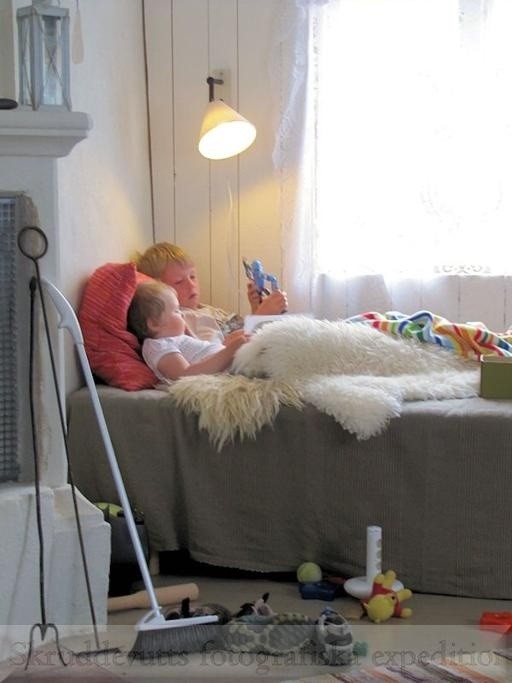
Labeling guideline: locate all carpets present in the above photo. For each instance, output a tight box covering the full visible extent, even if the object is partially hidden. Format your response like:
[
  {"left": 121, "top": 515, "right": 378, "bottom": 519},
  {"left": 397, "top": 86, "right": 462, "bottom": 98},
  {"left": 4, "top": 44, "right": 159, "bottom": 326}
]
[{"left": 280, "top": 647, "right": 512, "bottom": 683}]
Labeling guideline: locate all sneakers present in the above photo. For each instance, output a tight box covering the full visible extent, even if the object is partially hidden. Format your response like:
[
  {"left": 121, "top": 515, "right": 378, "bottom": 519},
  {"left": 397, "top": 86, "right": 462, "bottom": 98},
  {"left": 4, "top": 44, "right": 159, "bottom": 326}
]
[
  {"left": 312, "top": 607, "right": 352, "bottom": 665},
  {"left": 163, "top": 598, "right": 231, "bottom": 625},
  {"left": 222, "top": 592, "right": 311, "bottom": 656}
]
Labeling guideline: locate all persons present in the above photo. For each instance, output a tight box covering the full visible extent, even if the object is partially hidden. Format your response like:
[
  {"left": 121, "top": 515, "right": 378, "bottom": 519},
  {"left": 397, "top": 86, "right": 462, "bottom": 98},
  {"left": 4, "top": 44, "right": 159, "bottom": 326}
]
[
  {"left": 130, "top": 238, "right": 291, "bottom": 342},
  {"left": 128, "top": 279, "right": 251, "bottom": 383}
]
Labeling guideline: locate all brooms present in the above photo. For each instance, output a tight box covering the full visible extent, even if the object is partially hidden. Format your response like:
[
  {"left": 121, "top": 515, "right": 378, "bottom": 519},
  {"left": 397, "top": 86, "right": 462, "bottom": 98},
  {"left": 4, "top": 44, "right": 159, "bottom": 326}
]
[{"left": 39, "top": 276, "right": 227, "bottom": 656}]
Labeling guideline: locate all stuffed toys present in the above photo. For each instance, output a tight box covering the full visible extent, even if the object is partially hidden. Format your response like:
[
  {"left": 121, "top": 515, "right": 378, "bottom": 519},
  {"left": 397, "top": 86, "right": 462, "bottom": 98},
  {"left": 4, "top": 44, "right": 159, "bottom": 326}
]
[{"left": 365, "top": 568, "right": 414, "bottom": 622}]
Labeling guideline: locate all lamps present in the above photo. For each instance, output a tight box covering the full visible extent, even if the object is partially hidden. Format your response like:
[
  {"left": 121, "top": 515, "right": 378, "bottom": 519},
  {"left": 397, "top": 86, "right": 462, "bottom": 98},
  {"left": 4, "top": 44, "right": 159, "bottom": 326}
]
[{"left": 197, "top": 77, "right": 257, "bottom": 161}]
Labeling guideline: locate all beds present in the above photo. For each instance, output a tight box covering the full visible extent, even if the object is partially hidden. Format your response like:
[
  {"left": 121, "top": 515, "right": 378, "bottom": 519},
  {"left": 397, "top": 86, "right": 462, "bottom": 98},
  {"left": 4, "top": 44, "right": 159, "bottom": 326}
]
[{"left": 68, "top": 335, "right": 512, "bottom": 600}]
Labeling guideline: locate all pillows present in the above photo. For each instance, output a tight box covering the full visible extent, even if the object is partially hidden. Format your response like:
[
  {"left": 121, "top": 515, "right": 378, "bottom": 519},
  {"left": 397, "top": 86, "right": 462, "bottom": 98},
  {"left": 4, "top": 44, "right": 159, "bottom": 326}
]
[{"left": 81, "top": 262, "right": 160, "bottom": 394}]
